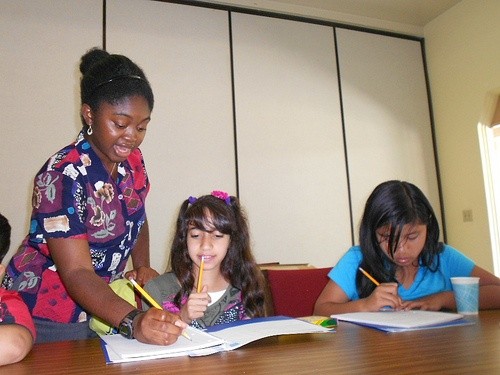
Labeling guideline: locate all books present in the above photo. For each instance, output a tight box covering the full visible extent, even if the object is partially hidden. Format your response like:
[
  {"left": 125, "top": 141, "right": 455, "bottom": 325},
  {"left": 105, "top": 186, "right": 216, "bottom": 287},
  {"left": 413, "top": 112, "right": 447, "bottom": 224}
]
[
  {"left": 101, "top": 326, "right": 225, "bottom": 360},
  {"left": 330, "top": 309, "right": 464, "bottom": 328}
]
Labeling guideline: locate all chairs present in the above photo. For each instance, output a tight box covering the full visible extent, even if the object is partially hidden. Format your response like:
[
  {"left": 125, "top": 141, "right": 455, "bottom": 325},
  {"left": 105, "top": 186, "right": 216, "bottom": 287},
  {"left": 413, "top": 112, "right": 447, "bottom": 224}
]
[{"left": 262, "top": 268, "right": 333, "bottom": 318}]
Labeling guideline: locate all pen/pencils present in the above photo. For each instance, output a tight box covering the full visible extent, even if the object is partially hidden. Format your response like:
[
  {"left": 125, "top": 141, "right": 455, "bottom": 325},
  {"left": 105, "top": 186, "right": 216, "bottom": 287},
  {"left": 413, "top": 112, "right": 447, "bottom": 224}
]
[
  {"left": 128, "top": 275, "right": 195, "bottom": 342},
  {"left": 196, "top": 255, "right": 205, "bottom": 294},
  {"left": 358, "top": 265, "right": 405, "bottom": 307}
]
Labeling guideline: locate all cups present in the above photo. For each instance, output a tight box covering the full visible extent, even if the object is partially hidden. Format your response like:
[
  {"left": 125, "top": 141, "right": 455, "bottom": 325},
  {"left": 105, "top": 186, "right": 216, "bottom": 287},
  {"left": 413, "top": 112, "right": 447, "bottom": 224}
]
[{"left": 450, "top": 277, "right": 480, "bottom": 315}]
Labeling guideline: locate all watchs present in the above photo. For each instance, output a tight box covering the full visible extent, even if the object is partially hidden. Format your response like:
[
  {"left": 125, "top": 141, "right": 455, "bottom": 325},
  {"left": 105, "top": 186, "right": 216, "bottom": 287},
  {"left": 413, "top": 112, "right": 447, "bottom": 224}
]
[{"left": 118, "top": 309, "right": 144, "bottom": 338}]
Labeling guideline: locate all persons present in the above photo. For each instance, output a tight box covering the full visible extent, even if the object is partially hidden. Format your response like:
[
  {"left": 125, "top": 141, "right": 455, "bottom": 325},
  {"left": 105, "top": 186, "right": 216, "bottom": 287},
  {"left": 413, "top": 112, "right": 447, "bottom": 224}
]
[
  {"left": 140, "top": 191, "right": 275, "bottom": 330},
  {"left": 0, "top": 214, "right": 37, "bottom": 366},
  {"left": 1, "top": 48, "right": 187, "bottom": 345},
  {"left": 313, "top": 180, "right": 500, "bottom": 318}
]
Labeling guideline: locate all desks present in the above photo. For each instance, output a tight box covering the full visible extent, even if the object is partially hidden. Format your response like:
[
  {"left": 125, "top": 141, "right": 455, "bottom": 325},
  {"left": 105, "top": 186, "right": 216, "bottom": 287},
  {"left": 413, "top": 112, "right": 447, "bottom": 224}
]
[{"left": 0, "top": 308, "right": 500, "bottom": 375}]
